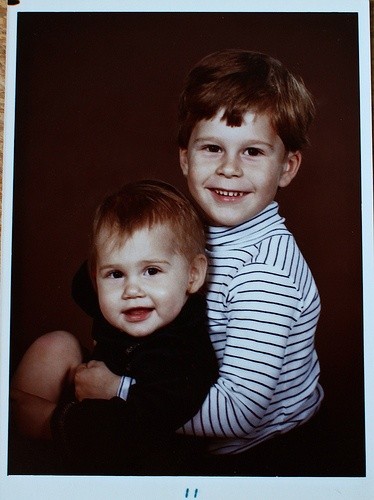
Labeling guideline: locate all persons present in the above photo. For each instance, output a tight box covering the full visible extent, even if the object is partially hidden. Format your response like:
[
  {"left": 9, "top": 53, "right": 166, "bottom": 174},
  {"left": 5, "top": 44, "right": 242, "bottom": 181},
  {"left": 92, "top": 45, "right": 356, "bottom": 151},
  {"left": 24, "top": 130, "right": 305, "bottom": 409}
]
[
  {"left": 64, "top": 47, "right": 332, "bottom": 477},
  {"left": 48, "top": 178, "right": 223, "bottom": 475}
]
